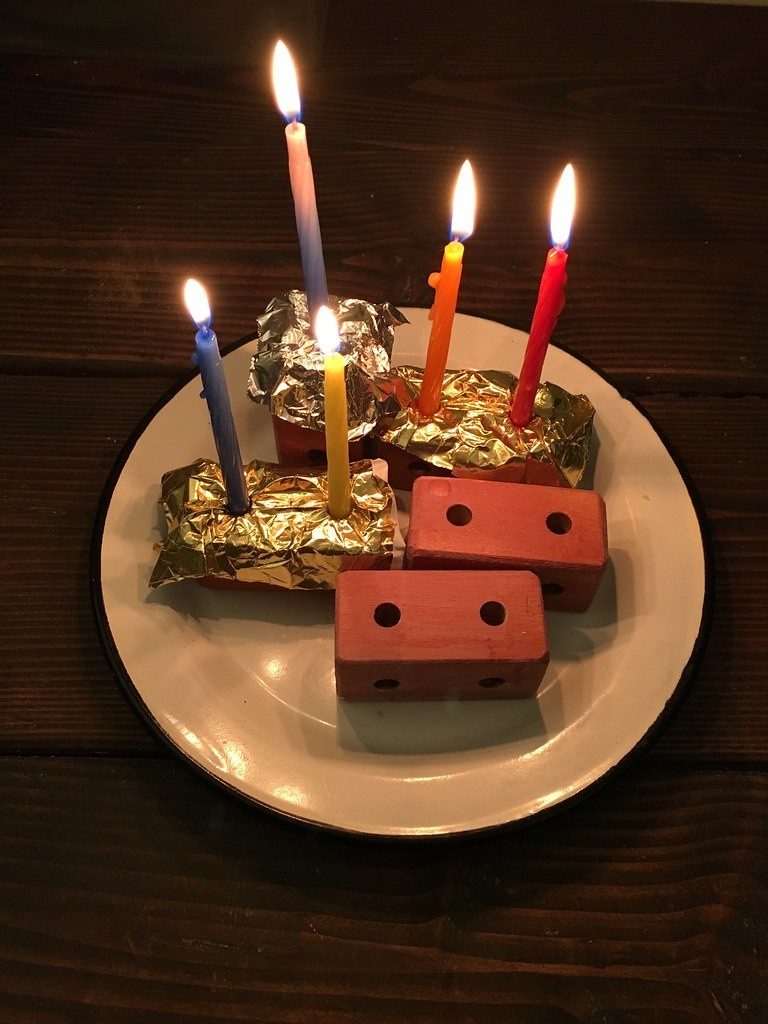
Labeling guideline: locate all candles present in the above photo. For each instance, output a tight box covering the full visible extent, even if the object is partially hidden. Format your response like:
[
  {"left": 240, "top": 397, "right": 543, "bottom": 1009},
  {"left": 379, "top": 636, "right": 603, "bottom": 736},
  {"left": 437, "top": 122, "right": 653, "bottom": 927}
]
[
  {"left": 509, "top": 162, "right": 577, "bottom": 426},
  {"left": 315, "top": 304, "right": 350, "bottom": 520},
  {"left": 180, "top": 279, "right": 249, "bottom": 514},
  {"left": 270, "top": 39, "right": 328, "bottom": 339},
  {"left": 417, "top": 159, "right": 476, "bottom": 416}
]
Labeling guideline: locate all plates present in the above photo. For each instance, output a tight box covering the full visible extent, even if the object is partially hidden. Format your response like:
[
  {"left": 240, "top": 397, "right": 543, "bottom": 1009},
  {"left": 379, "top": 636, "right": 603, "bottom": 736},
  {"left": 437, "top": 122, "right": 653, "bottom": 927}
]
[{"left": 95, "top": 306, "right": 709, "bottom": 838}]
